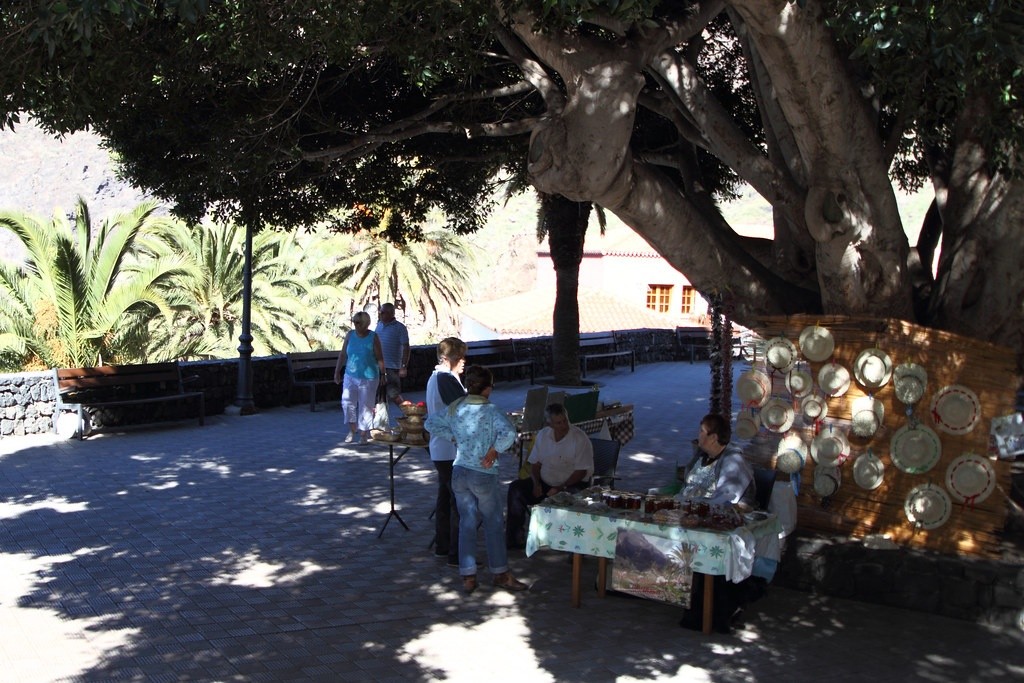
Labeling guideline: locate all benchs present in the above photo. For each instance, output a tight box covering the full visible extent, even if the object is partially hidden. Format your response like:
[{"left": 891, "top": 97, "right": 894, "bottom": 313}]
[
  {"left": 286, "top": 350, "right": 389, "bottom": 413},
  {"left": 543, "top": 331, "right": 636, "bottom": 379},
  {"left": 57, "top": 359, "right": 206, "bottom": 441}
]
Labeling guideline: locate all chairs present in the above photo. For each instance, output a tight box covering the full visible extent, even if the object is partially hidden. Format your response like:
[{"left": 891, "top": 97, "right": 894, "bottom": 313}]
[
  {"left": 587, "top": 435, "right": 625, "bottom": 491},
  {"left": 747, "top": 468, "right": 784, "bottom": 603}
]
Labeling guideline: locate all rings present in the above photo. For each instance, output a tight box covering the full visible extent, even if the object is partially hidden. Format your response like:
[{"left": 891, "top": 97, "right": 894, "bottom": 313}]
[
  {"left": 491, "top": 461, "right": 494, "bottom": 464},
  {"left": 488, "top": 462, "right": 490, "bottom": 464}
]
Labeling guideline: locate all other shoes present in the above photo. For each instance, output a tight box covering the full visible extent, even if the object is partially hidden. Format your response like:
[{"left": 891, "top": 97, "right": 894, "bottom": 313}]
[
  {"left": 491, "top": 570, "right": 526, "bottom": 590},
  {"left": 446, "top": 554, "right": 483, "bottom": 568},
  {"left": 344, "top": 430, "right": 354, "bottom": 442},
  {"left": 359, "top": 435, "right": 367, "bottom": 445},
  {"left": 462, "top": 575, "right": 476, "bottom": 593}
]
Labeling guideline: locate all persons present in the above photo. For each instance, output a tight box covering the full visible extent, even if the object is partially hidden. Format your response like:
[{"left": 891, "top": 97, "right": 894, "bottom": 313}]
[
  {"left": 375, "top": 303, "right": 411, "bottom": 406},
  {"left": 507, "top": 404, "right": 595, "bottom": 551},
  {"left": 423, "top": 366, "right": 527, "bottom": 594},
  {"left": 426, "top": 337, "right": 484, "bottom": 569},
  {"left": 673, "top": 413, "right": 757, "bottom": 511},
  {"left": 334, "top": 311, "right": 385, "bottom": 446}
]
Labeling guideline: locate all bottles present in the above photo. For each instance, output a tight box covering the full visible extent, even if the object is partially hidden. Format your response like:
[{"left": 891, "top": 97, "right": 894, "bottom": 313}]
[
  {"left": 684, "top": 498, "right": 691, "bottom": 511},
  {"left": 645, "top": 495, "right": 681, "bottom": 513},
  {"left": 600, "top": 490, "right": 641, "bottom": 509}
]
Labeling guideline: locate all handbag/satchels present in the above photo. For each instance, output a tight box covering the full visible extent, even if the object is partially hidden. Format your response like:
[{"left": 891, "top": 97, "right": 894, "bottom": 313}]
[
  {"left": 373, "top": 383, "right": 391, "bottom": 432},
  {"left": 497, "top": 452, "right": 520, "bottom": 486}
]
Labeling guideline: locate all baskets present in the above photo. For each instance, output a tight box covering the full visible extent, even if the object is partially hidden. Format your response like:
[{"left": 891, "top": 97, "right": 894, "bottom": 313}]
[{"left": 399, "top": 404, "right": 428, "bottom": 415}]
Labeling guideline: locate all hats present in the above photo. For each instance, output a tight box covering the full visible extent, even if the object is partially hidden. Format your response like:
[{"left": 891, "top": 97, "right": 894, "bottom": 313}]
[{"left": 736, "top": 325, "right": 995, "bottom": 530}]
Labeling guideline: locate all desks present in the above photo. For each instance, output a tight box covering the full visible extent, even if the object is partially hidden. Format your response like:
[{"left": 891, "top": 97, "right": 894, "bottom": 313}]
[
  {"left": 462, "top": 338, "right": 535, "bottom": 395},
  {"left": 366, "top": 401, "right": 637, "bottom": 538},
  {"left": 526, "top": 490, "right": 771, "bottom": 634},
  {"left": 677, "top": 326, "right": 757, "bottom": 365}
]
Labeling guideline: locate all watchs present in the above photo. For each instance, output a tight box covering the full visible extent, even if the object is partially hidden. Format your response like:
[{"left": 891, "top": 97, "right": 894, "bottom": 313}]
[
  {"left": 562, "top": 485, "right": 567, "bottom": 490},
  {"left": 381, "top": 373, "right": 387, "bottom": 375},
  {"left": 401, "top": 364, "right": 408, "bottom": 367}
]
